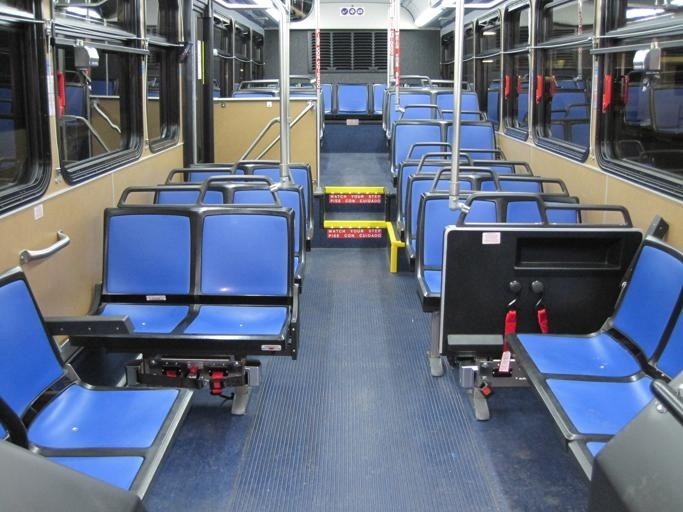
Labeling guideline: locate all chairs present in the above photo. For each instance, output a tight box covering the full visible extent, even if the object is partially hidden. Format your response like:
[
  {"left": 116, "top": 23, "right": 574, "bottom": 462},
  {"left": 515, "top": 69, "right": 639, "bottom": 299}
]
[
  {"left": 0, "top": 163, "right": 312, "bottom": 504},
  {"left": 485, "top": 75, "right": 681, "bottom": 151},
  {"left": 230, "top": 78, "right": 679, "bottom": 484},
  {"left": 0, "top": 76, "right": 220, "bottom": 131}
]
